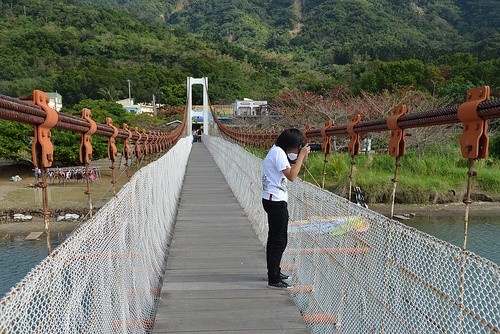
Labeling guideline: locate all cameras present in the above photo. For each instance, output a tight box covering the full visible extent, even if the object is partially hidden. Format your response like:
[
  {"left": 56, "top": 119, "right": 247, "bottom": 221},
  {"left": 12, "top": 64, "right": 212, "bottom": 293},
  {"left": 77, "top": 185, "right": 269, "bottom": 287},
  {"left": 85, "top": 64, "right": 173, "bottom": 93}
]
[{"left": 302, "top": 140, "right": 322, "bottom": 151}]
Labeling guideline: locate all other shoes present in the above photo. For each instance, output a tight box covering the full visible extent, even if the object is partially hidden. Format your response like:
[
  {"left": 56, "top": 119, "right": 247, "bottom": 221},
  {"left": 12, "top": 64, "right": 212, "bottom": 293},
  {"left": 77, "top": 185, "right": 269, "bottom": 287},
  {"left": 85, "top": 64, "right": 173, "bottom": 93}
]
[
  {"left": 268, "top": 281, "right": 294, "bottom": 291},
  {"left": 267, "top": 272, "right": 290, "bottom": 281}
]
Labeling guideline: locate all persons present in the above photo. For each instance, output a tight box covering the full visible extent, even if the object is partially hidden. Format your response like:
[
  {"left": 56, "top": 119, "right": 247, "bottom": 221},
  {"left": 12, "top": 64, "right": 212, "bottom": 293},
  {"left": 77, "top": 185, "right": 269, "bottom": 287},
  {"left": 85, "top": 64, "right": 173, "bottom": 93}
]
[
  {"left": 262, "top": 128, "right": 311, "bottom": 288},
  {"left": 192, "top": 128, "right": 202, "bottom": 143}
]
